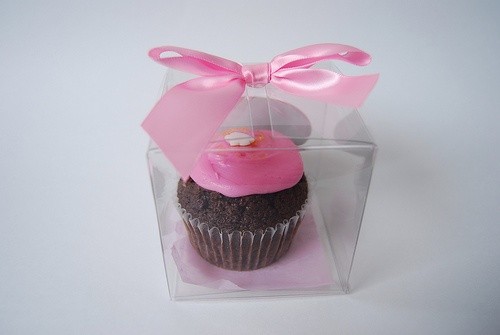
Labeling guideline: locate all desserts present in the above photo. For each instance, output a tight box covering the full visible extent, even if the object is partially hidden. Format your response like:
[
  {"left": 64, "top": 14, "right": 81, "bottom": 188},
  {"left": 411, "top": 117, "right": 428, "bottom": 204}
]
[{"left": 176, "top": 129, "right": 308, "bottom": 272}]
